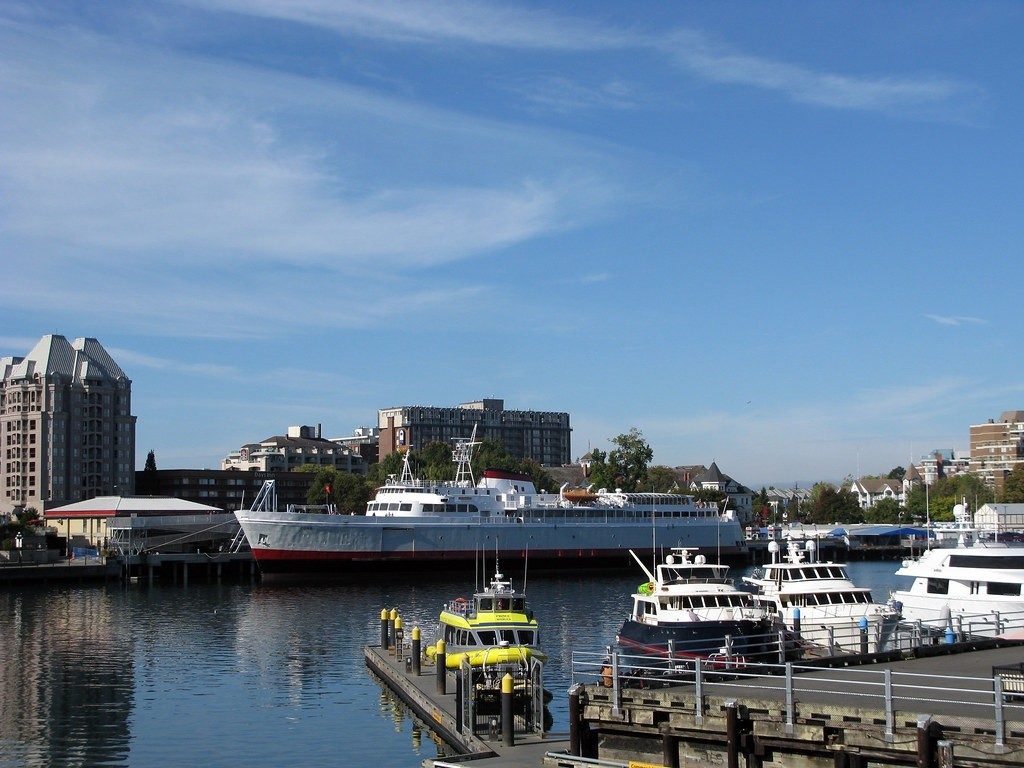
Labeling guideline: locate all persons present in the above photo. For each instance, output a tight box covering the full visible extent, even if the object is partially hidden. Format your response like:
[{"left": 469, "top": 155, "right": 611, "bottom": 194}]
[
  {"left": 330, "top": 510, "right": 375, "bottom": 516},
  {"left": 746, "top": 595, "right": 754, "bottom": 608},
  {"left": 600, "top": 661, "right": 613, "bottom": 688}
]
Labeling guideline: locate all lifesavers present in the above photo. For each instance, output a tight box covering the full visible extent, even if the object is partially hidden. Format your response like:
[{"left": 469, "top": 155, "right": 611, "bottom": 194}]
[{"left": 454, "top": 596, "right": 468, "bottom": 611}]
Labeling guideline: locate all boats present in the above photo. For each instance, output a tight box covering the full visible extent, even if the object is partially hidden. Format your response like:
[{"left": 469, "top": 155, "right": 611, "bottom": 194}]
[
  {"left": 474, "top": 648, "right": 550, "bottom": 710},
  {"left": 425, "top": 536, "right": 548, "bottom": 669},
  {"left": 614, "top": 477, "right": 1024, "bottom": 686},
  {"left": 563, "top": 491, "right": 600, "bottom": 502}
]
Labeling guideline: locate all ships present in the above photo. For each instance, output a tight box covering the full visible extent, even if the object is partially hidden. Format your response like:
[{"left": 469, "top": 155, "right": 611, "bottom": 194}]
[{"left": 232, "top": 418, "right": 749, "bottom": 570}]
